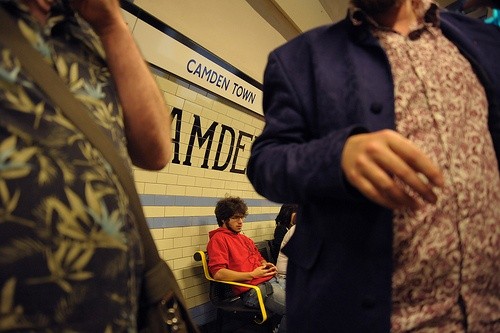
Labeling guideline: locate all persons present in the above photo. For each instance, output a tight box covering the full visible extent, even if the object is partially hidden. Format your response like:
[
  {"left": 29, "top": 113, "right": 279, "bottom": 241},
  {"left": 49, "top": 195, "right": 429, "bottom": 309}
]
[
  {"left": 1, "top": 0, "right": 175, "bottom": 333},
  {"left": 246, "top": 0, "right": 500, "bottom": 333},
  {"left": 207, "top": 196, "right": 296, "bottom": 333}
]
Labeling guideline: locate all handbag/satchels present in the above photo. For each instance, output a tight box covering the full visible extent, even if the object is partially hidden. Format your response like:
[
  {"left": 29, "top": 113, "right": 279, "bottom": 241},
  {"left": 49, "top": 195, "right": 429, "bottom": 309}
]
[{"left": 138, "top": 259, "right": 192, "bottom": 333}]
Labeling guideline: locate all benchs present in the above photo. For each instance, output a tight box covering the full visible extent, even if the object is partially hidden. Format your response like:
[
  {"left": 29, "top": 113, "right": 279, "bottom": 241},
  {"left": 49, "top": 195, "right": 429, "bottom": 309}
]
[{"left": 193, "top": 240, "right": 274, "bottom": 333}]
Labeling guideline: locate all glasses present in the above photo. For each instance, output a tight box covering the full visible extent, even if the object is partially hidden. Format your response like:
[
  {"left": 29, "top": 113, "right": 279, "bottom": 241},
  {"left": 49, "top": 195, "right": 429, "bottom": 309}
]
[{"left": 230, "top": 216, "right": 246, "bottom": 222}]
